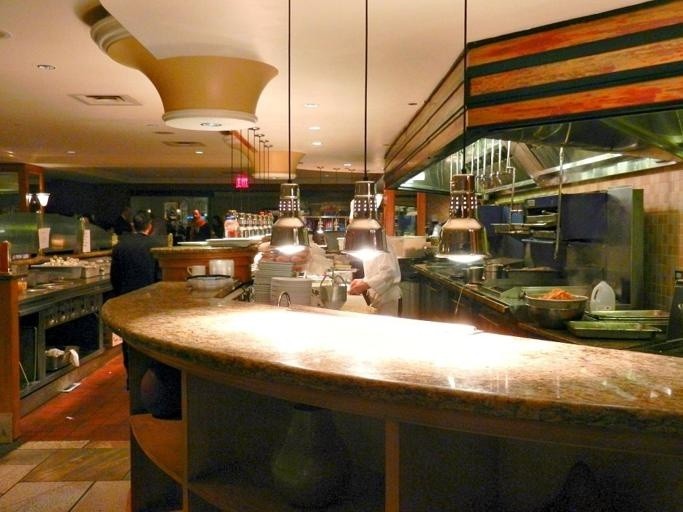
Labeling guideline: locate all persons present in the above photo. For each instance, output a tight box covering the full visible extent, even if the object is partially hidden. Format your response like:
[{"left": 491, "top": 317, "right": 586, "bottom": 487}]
[
  {"left": 185, "top": 208, "right": 211, "bottom": 242},
  {"left": 111, "top": 203, "right": 133, "bottom": 236},
  {"left": 165, "top": 212, "right": 185, "bottom": 247},
  {"left": 109, "top": 210, "right": 165, "bottom": 393},
  {"left": 343, "top": 209, "right": 404, "bottom": 318},
  {"left": 211, "top": 215, "right": 224, "bottom": 239}
]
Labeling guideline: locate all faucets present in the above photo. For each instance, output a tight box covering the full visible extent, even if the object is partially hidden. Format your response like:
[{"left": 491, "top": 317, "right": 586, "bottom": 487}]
[{"left": 277, "top": 291, "right": 291, "bottom": 307}]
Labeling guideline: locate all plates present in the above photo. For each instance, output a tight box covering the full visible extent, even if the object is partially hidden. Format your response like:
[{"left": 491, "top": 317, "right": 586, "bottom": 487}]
[{"left": 253, "top": 260, "right": 312, "bottom": 307}]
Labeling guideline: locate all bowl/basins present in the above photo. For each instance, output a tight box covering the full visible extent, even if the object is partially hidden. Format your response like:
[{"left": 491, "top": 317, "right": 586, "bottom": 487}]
[{"left": 524, "top": 293, "right": 589, "bottom": 328}]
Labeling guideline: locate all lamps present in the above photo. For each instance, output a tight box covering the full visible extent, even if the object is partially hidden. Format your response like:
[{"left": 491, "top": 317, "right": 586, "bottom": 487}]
[
  {"left": 225, "top": 127, "right": 273, "bottom": 237},
  {"left": 270, "top": 1, "right": 310, "bottom": 257},
  {"left": 341, "top": 0, "right": 390, "bottom": 261},
  {"left": 435, "top": 1, "right": 487, "bottom": 265},
  {"left": 305, "top": 103, "right": 324, "bottom": 149}
]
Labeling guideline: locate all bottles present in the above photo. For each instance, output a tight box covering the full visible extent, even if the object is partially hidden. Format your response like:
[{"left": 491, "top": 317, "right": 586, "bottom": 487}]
[{"left": 222, "top": 209, "right": 274, "bottom": 239}]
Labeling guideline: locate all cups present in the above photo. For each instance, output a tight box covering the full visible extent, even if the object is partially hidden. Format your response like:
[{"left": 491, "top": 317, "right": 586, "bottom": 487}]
[
  {"left": 336, "top": 237, "right": 345, "bottom": 251},
  {"left": 185, "top": 265, "right": 206, "bottom": 278}
]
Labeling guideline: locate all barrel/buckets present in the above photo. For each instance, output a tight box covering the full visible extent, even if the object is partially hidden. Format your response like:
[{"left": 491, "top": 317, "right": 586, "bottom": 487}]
[{"left": 590, "top": 280, "right": 615, "bottom": 311}]
